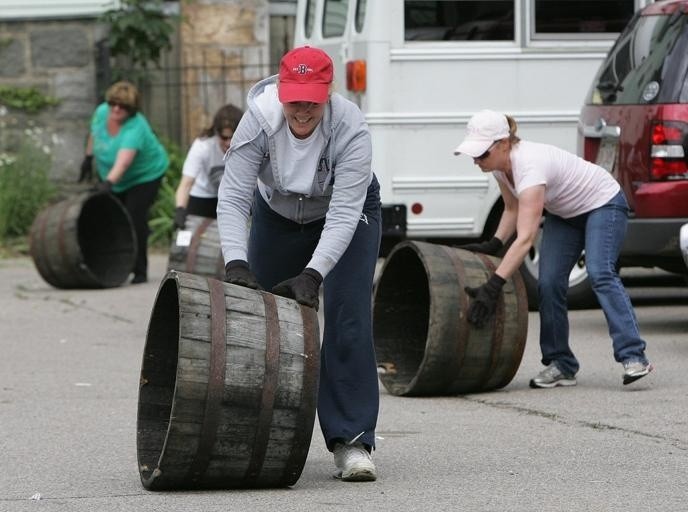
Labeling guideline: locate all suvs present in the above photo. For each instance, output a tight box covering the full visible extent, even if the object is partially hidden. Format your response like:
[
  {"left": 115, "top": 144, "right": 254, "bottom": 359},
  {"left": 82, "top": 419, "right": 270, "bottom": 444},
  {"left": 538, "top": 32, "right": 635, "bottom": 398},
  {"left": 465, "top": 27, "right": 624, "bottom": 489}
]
[{"left": 575, "top": 0, "right": 687, "bottom": 276}]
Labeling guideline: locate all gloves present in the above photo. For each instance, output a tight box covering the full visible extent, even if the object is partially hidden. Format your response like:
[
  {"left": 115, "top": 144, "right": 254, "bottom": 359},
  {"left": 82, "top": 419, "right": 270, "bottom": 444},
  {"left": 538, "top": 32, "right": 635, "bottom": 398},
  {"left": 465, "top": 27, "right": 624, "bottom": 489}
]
[
  {"left": 77, "top": 154, "right": 95, "bottom": 184},
  {"left": 451, "top": 235, "right": 506, "bottom": 259},
  {"left": 463, "top": 272, "right": 508, "bottom": 326},
  {"left": 271, "top": 267, "right": 324, "bottom": 312},
  {"left": 89, "top": 179, "right": 115, "bottom": 193},
  {"left": 172, "top": 207, "right": 190, "bottom": 231},
  {"left": 222, "top": 259, "right": 264, "bottom": 290}
]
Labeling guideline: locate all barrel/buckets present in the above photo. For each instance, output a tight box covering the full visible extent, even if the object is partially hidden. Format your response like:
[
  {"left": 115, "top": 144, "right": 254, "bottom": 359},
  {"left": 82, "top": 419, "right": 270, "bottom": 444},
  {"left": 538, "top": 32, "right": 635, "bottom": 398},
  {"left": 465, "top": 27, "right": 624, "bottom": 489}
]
[
  {"left": 164, "top": 213, "right": 221, "bottom": 278},
  {"left": 371, "top": 238, "right": 530, "bottom": 396},
  {"left": 136, "top": 269, "right": 321, "bottom": 492},
  {"left": 26, "top": 191, "right": 141, "bottom": 289}
]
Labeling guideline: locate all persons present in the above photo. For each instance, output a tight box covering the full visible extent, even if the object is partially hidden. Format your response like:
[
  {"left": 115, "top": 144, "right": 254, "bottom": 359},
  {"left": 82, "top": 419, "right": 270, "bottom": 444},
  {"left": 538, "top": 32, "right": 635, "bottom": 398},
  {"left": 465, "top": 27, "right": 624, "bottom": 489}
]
[
  {"left": 78, "top": 81, "right": 170, "bottom": 284},
  {"left": 171, "top": 103, "right": 244, "bottom": 230},
  {"left": 453, "top": 110, "right": 653, "bottom": 388},
  {"left": 216, "top": 46, "right": 382, "bottom": 485}
]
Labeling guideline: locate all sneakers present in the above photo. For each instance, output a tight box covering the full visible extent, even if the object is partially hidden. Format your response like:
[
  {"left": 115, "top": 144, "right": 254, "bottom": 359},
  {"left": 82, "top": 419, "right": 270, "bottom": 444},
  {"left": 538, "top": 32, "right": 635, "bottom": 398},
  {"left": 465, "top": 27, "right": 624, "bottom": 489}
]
[
  {"left": 622, "top": 353, "right": 653, "bottom": 388},
  {"left": 528, "top": 359, "right": 579, "bottom": 390},
  {"left": 331, "top": 443, "right": 379, "bottom": 483}
]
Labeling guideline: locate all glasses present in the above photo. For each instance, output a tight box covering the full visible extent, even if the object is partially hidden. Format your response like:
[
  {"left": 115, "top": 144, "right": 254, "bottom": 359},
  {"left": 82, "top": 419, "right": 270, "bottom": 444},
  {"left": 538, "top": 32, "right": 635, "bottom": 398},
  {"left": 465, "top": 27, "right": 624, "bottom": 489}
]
[
  {"left": 108, "top": 100, "right": 129, "bottom": 110},
  {"left": 218, "top": 134, "right": 232, "bottom": 141},
  {"left": 476, "top": 140, "right": 501, "bottom": 159}
]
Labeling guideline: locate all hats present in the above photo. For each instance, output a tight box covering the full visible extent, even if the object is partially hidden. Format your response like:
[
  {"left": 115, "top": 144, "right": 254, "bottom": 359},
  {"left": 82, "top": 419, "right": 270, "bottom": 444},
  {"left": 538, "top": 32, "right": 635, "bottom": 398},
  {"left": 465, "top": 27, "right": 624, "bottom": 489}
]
[
  {"left": 453, "top": 109, "right": 511, "bottom": 158},
  {"left": 276, "top": 45, "right": 335, "bottom": 106}
]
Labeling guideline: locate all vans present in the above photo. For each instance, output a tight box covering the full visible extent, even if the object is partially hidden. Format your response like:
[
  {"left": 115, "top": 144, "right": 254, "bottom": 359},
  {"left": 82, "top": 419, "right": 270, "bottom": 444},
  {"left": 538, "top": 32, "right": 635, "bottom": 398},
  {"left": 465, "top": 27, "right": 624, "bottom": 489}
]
[{"left": 292, "top": 0, "right": 659, "bottom": 310}]
